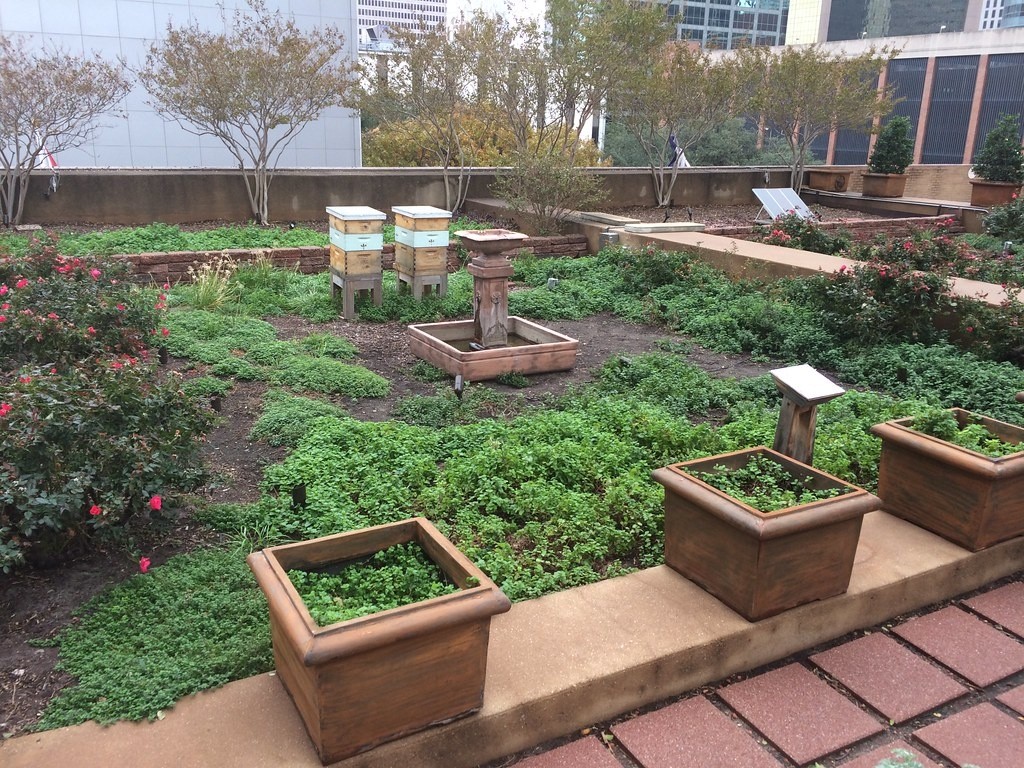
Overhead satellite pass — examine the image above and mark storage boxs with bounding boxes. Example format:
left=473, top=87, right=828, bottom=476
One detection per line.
left=394, top=213, right=448, bottom=277
left=328, top=214, right=385, bottom=279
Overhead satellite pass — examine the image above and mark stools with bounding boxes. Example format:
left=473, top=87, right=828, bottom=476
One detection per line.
left=329, top=267, right=382, bottom=320
left=393, top=263, right=448, bottom=302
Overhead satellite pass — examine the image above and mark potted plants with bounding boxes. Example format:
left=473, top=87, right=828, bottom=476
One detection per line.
left=649, top=446, right=887, bottom=623
left=246, top=516, right=510, bottom=766
left=968, top=113, right=1024, bottom=207
left=870, top=407, right=1024, bottom=552
left=862, top=116, right=914, bottom=197
